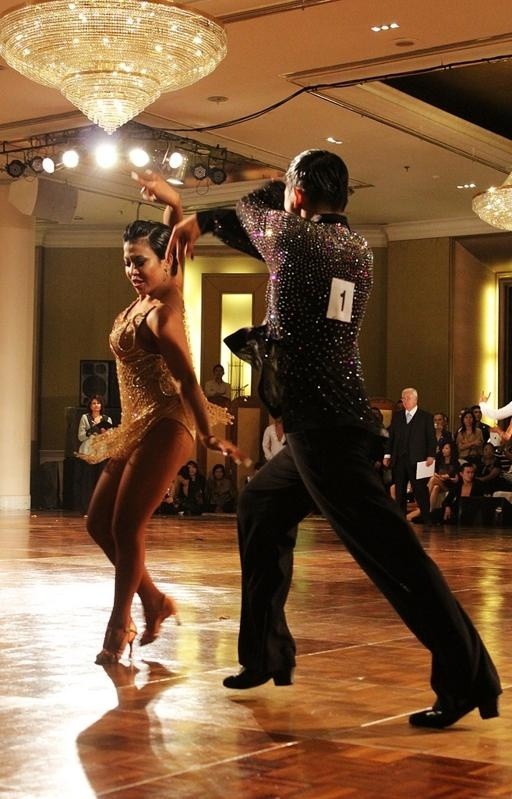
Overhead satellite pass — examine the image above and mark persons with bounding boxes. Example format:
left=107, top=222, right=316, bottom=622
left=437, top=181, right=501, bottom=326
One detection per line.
left=262, top=418, right=287, bottom=462
left=165, top=148, right=502, bottom=730
left=368, top=388, right=512, bottom=530
left=78, top=394, right=114, bottom=443
left=201, top=365, right=228, bottom=408
left=85, top=158, right=229, bottom=664
left=153, top=460, right=233, bottom=520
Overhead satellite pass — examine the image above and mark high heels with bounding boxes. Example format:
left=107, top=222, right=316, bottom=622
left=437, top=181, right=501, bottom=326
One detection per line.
left=95, top=594, right=182, bottom=664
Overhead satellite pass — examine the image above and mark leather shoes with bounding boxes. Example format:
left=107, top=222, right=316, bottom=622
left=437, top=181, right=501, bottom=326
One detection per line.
left=223, top=664, right=295, bottom=689
left=410, top=694, right=499, bottom=728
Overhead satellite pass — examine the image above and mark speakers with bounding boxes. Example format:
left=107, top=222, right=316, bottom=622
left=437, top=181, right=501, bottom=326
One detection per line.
left=79, top=359, right=120, bottom=408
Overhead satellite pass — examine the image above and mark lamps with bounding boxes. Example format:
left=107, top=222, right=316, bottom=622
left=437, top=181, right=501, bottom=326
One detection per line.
left=0, top=121, right=226, bottom=195
left=471, top=171, right=512, bottom=231
left=0, top=0, right=227, bottom=135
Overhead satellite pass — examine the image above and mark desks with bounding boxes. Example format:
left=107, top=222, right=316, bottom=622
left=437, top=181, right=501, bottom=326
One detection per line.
left=40, top=450, right=65, bottom=508
left=458, top=496, right=512, bottom=529
